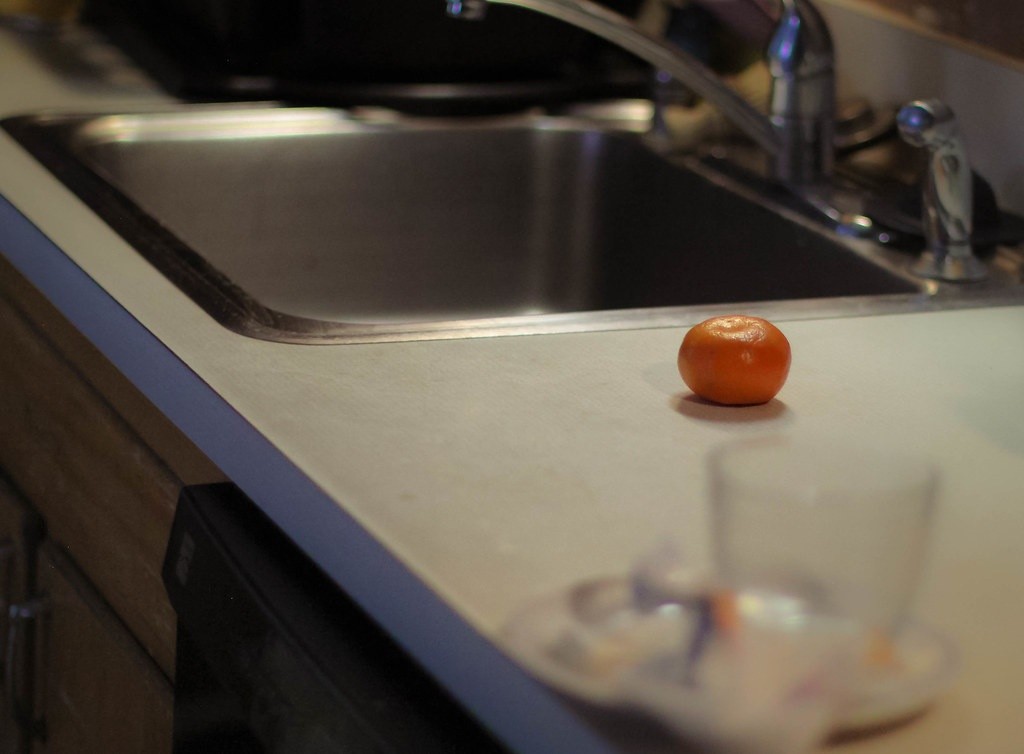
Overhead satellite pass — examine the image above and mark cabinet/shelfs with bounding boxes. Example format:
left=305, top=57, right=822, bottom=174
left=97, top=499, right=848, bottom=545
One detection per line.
left=0, top=254, right=515, bottom=754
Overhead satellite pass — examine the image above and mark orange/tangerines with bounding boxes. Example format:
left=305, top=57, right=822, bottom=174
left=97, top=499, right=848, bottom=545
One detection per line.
left=677, top=314, right=792, bottom=406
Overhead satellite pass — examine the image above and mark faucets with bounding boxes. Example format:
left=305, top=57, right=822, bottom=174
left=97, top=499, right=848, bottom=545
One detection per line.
left=439, top=0, right=849, bottom=205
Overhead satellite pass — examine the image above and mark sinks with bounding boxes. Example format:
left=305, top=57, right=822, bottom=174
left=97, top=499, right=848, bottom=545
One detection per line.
left=0, top=107, right=946, bottom=346
